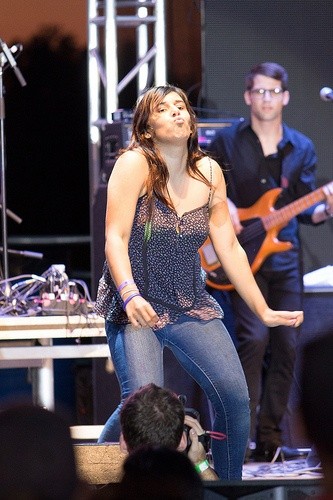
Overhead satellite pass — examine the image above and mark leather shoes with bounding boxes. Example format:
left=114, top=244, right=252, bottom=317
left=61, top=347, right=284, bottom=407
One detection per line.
left=251, top=445, right=303, bottom=461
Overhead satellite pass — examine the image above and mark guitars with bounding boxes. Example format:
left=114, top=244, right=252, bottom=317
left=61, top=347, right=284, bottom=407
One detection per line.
left=197, top=180, right=333, bottom=292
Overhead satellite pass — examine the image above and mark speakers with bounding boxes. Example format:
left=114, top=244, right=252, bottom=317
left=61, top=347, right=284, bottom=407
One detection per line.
left=288, top=285, right=333, bottom=453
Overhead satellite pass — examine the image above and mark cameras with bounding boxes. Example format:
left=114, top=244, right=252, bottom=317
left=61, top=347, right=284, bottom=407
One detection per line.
left=178, top=395, right=201, bottom=457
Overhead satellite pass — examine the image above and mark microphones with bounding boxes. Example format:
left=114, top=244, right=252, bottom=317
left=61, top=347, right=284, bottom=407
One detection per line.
left=320, top=87, right=333, bottom=102
left=0, top=43, right=21, bottom=67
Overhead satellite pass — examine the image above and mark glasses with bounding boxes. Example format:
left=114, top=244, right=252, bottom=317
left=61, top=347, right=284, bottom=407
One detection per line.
left=249, top=87, right=284, bottom=94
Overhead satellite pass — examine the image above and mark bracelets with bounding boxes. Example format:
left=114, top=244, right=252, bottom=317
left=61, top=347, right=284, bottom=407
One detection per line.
left=122, top=292, right=143, bottom=312
left=120, top=288, right=140, bottom=299
left=324, top=204, right=333, bottom=218
left=117, top=280, right=137, bottom=293
left=192, top=460, right=210, bottom=474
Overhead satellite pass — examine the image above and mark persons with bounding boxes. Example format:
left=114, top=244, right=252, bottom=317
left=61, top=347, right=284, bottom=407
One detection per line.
left=0, top=328, right=333, bottom=500
left=207, top=63, right=333, bottom=464
left=91, top=85, right=304, bottom=480
